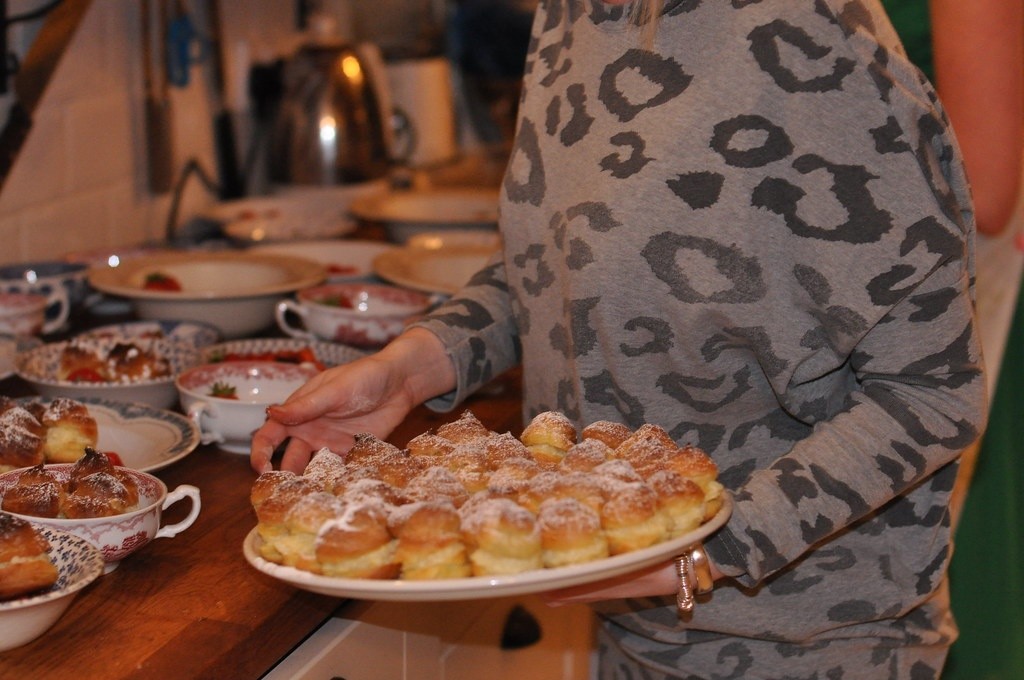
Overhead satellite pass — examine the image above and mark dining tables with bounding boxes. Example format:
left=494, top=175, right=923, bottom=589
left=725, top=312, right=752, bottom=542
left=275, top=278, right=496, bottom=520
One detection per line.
left=0, top=294, right=598, bottom=680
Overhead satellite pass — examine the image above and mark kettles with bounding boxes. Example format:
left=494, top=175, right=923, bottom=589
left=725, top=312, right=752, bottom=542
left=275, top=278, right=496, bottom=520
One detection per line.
left=277, top=37, right=390, bottom=188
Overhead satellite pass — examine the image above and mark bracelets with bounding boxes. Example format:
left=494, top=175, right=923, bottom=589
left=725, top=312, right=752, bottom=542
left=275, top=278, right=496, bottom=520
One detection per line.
left=673, top=556, right=695, bottom=611
left=689, top=545, right=713, bottom=595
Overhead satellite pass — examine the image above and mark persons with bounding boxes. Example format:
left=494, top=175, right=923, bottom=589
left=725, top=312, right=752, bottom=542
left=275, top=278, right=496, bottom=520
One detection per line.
left=251, top=0, right=986, bottom=679
left=879, top=0, right=1024, bottom=680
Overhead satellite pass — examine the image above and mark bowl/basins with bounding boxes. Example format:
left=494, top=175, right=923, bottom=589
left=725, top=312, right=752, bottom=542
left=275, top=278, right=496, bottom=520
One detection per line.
left=12, top=395, right=202, bottom=473
left=347, top=186, right=499, bottom=245
left=274, top=282, right=430, bottom=351
left=0, top=261, right=90, bottom=338
left=199, top=338, right=370, bottom=372
left=76, top=318, right=221, bottom=348
left=0, top=521, right=105, bottom=653
left=0, top=463, right=202, bottom=576
left=196, top=180, right=390, bottom=243
left=11, top=336, right=201, bottom=411
left=86, top=249, right=330, bottom=340
left=172, top=361, right=319, bottom=455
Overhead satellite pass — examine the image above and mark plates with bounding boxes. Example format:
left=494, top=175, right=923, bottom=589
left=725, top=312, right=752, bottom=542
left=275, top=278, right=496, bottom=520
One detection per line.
left=372, top=243, right=500, bottom=296
left=242, top=493, right=731, bottom=601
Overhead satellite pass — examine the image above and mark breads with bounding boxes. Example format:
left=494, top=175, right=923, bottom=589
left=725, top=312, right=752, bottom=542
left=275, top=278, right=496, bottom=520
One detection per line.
left=0, top=396, right=151, bottom=600
left=249, top=410, right=724, bottom=581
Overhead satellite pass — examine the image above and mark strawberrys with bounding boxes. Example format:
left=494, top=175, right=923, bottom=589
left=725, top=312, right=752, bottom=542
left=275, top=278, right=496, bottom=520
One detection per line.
left=142, top=272, right=181, bottom=291
left=207, top=382, right=239, bottom=400
left=65, top=369, right=104, bottom=383
